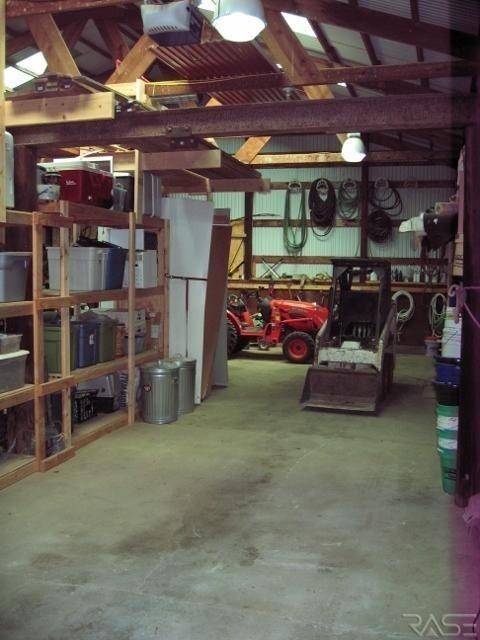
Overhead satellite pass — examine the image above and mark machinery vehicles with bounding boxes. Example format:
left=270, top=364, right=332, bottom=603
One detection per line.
left=227, top=257, right=399, bottom=412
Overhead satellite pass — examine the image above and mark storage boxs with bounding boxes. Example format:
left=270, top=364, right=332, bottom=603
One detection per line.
left=0, top=333, right=23, bottom=354
left=36, top=161, right=134, bottom=212
left=44, top=308, right=157, bottom=373
left=76, top=373, right=122, bottom=414
left=46, top=247, right=158, bottom=291
left=0, top=252, right=32, bottom=302
left=0, top=350, right=30, bottom=393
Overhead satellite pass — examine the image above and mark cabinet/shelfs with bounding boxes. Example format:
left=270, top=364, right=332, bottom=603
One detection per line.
left=0, top=210, right=169, bottom=490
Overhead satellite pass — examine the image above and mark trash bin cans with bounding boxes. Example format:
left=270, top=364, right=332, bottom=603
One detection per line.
left=139, top=361, right=179, bottom=425
left=159, top=358, right=197, bottom=414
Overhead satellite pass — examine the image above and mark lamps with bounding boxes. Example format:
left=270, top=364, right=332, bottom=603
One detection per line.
left=211, top=0, right=267, bottom=43
left=341, top=133, right=367, bottom=163
